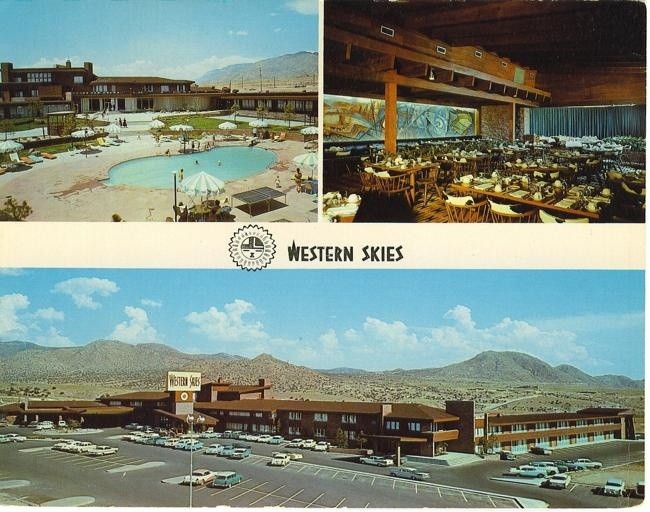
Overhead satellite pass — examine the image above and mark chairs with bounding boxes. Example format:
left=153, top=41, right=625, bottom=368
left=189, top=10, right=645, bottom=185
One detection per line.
left=275, top=132, right=286, bottom=142
left=97, top=136, right=120, bottom=147
left=334, top=145, right=646, bottom=223
left=172, top=205, right=231, bottom=222
left=9, top=152, right=57, bottom=166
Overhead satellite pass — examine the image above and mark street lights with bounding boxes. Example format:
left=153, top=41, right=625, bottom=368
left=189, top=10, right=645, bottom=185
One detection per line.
left=171, top=170, right=178, bottom=222
left=84, top=128, right=88, bottom=157
left=186, top=414, right=205, bottom=507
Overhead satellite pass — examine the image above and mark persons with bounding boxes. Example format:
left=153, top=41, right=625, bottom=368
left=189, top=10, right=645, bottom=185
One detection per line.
left=275, top=176, right=282, bottom=188
left=294, top=168, right=302, bottom=193
left=179, top=201, right=188, bottom=213
left=253, top=128, right=258, bottom=136
left=178, top=169, right=184, bottom=184
left=209, top=200, right=221, bottom=221
left=164, top=149, right=170, bottom=157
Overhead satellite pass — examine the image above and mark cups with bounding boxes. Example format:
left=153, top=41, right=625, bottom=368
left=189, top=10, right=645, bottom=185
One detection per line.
left=461, top=171, right=612, bottom=210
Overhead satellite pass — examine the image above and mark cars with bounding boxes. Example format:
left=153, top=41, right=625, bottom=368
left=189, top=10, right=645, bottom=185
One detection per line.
left=495, top=450, right=516, bottom=460
left=200, top=431, right=331, bottom=466
left=604, top=479, right=625, bottom=496
left=184, top=468, right=241, bottom=488
left=29, top=421, right=66, bottom=430
left=54, top=440, right=118, bottom=456
left=0, top=433, right=26, bottom=443
left=123, top=423, right=204, bottom=451
left=531, top=446, right=552, bottom=455
left=508, top=458, right=602, bottom=488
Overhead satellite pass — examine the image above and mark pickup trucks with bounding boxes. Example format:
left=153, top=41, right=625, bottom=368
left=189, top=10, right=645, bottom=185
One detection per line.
left=359, top=456, right=394, bottom=467
left=390, top=468, right=430, bottom=480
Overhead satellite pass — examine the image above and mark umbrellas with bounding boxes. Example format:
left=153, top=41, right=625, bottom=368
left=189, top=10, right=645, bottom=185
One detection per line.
left=218, top=121, right=238, bottom=137
left=302, top=127, right=318, bottom=142
left=177, top=170, right=226, bottom=222
left=168, top=124, right=194, bottom=133
left=293, top=152, right=318, bottom=180
left=0, top=140, right=25, bottom=154
left=70, top=129, right=96, bottom=153
left=149, top=119, right=166, bottom=130
left=104, top=123, right=121, bottom=142
left=249, top=118, right=271, bottom=130
